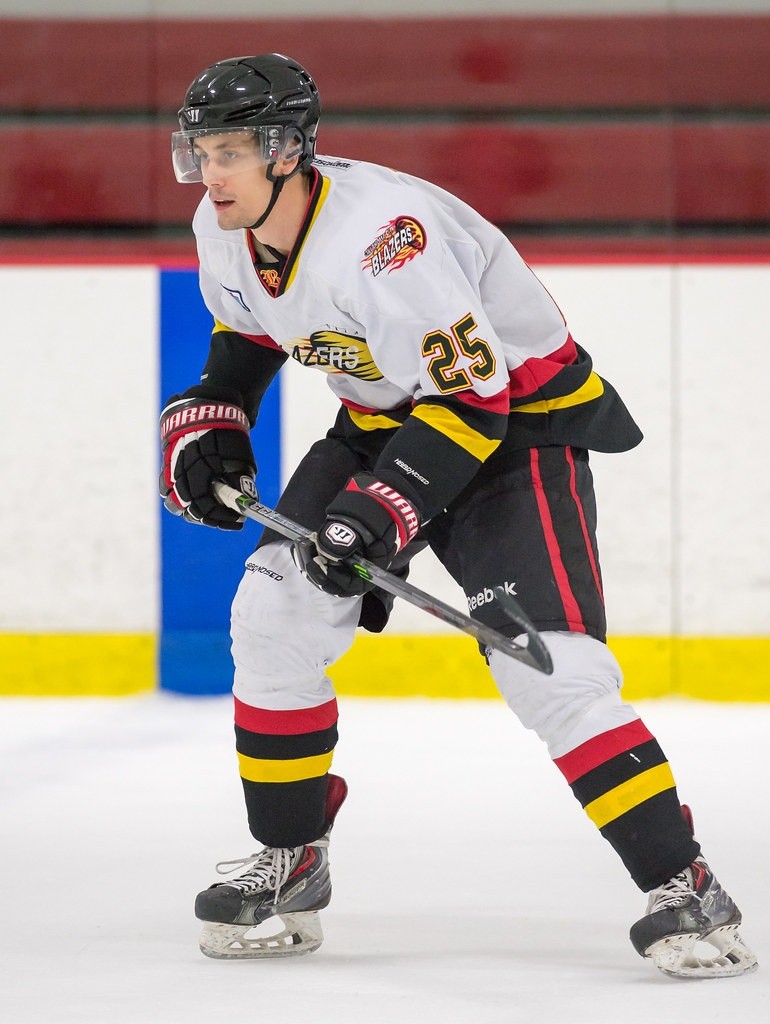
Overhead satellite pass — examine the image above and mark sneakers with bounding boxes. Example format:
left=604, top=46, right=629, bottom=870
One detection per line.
left=627, top=856, right=760, bottom=979
left=195, top=846, right=333, bottom=960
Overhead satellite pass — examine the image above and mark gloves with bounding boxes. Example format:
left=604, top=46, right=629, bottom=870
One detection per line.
left=289, top=470, right=423, bottom=599
left=159, top=385, right=260, bottom=532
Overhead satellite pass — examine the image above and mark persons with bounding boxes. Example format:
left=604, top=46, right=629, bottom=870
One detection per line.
left=157, top=54, right=759, bottom=981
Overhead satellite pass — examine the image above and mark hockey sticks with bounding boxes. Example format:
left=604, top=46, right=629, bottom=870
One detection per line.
left=213, top=482, right=557, bottom=680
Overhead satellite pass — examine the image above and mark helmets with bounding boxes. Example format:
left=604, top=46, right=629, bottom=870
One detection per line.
left=178, top=53, right=323, bottom=184
